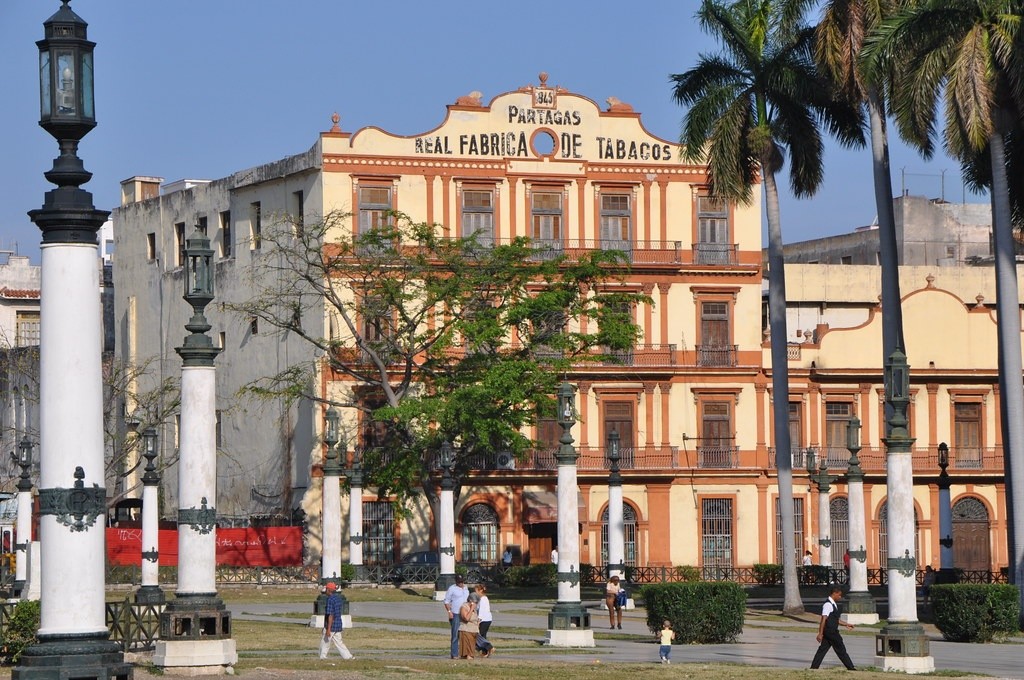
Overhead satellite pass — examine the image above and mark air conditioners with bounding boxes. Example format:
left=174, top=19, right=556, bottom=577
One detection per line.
left=496, top=451, right=515, bottom=469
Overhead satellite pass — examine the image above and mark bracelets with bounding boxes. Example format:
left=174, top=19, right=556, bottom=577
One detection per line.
left=448, top=610, right=451, bottom=613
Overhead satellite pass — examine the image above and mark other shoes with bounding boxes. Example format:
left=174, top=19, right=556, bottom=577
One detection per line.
left=451, top=655, right=459, bottom=660
left=617, top=623, right=623, bottom=630
left=610, top=625, right=614, bottom=629
left=661, top=657, right=667, bottom=664
left=487, top=647, right=496, bottom=657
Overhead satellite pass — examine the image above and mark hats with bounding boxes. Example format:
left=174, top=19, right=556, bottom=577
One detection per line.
left=455, top=574, right=466, bottom=583
left=327, top=583, right=336, bottom=591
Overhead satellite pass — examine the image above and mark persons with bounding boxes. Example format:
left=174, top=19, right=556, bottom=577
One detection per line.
left=809, top=586, right=857, bottom=671
left=503, top=545, right=513, bottom=566
left=921, top=565, right=936, bottom=608
left=657, top=620, right=676, bottom=664
left=550, top=545, right=559, bottom=565
left=802, top=551, right=813, bottom=565
left=319, top=582, right=356, bottom=660
left=843, top=549, right=850, bottom=585
left=443, top=574, right=496, bottom=659
left=606, top=575, right=623, bottom=629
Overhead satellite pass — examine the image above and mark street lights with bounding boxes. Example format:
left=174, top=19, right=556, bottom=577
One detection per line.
left=309, top=402, right=352, bottom=629
left=541, top=371, right=596, bottom=647
left=11, top=0, right=134, bottom=680
left=873, top=334, right=935, bottom=676
left=839, top=414, right=880, bottom=624
left=600, top=427, right=634, bottom=610
left=936, top=442, right=957, bottom=585
left=135, top=419, right=166, bottom=606
left=433, top=437, right=456, bottom=601
left=152, top=224, right=240, bottom=667
left=7, top=436, right=34, bottom=598
